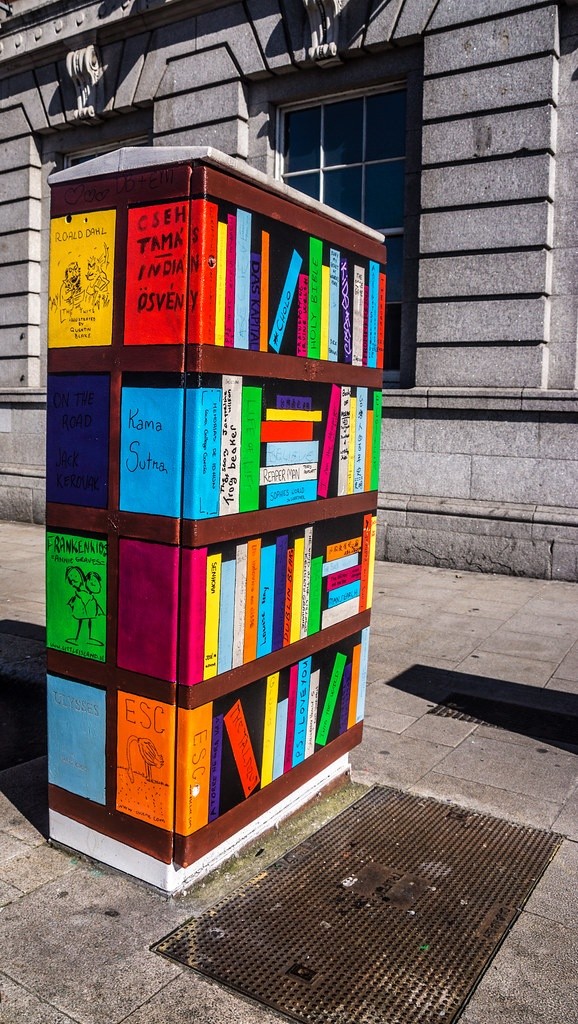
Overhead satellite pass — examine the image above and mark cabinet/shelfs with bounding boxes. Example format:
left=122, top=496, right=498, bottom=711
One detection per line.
left=47, top=145, right=386, bottom=896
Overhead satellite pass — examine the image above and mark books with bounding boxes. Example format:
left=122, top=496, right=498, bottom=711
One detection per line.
left=46, top=514, right=378, bottom=685
left=48, top=627, right=370, bottom=836
left=47, top=374, right=383, bottom=520
left=49, top=196, right=387, bottom=368
